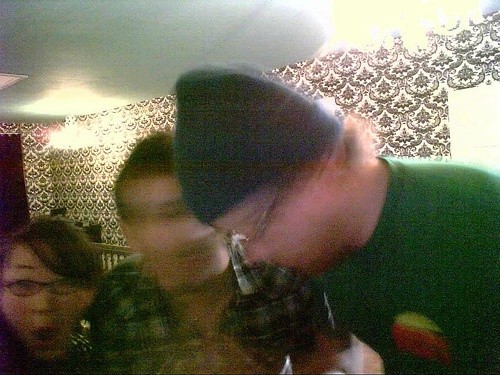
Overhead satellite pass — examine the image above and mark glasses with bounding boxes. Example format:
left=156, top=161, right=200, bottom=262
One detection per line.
left=3, top=276, right=88, bottom=297
left=222, top=186, right=287, bottom=256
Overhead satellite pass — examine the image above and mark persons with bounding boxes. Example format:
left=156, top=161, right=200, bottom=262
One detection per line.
left=88, top=129, right=366, bottom=375
left=174, top=66, right=499, bottom=374
left=1, top=218, right=102, bottom=375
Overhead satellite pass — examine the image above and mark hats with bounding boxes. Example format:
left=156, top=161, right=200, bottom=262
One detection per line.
left=175, top=66, right=341, bottom=224
